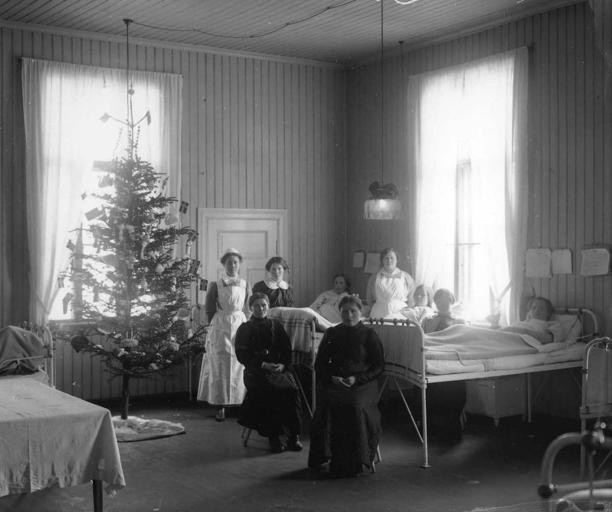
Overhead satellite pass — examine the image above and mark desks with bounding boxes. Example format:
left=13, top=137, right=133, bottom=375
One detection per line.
left=0, top=376, right=126, bottom=512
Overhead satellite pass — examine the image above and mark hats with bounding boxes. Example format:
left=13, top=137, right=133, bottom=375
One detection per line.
left=220, top=248, right=243, bottom=264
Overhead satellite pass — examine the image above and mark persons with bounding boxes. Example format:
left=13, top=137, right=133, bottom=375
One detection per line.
left=251, top=256, right=294, bottom=308
left=308, top=273, right=353, bottom=322
left=306, top=294, right=385, bottom=478
left=194, top=247, right=253, bottom=421
left=401, top=281, right=434, bottom=322
left=364, top=247, right=414, bottom=318
left=496, top=295, right=564, bottom=344
left=414, top=289, right=467, bottom=446
left=234, top=292, right=305, bottom=454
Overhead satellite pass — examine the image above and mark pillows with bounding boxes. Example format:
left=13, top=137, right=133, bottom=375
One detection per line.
left=547, top=313, right=581, bottom=347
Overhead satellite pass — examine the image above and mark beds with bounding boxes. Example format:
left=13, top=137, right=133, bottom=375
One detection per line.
left=268, top=307, right=344, bottom=421
left=0, top=321, right=54, bottom=389
left=357, top=306, right=598, bottom=470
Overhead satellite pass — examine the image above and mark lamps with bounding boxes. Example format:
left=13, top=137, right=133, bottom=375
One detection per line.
left=365, top=0, right=403, bottom=221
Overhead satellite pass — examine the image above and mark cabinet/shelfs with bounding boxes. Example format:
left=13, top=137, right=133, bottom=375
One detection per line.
left=465, top=374, right=529, bottom=429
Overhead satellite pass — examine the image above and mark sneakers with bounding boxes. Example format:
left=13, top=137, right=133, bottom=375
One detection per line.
left=269, top=437, right=286, bottom=452
left=287, top=438, right=302, bottom=451
left=216, top=410, right=226, bottom=421
